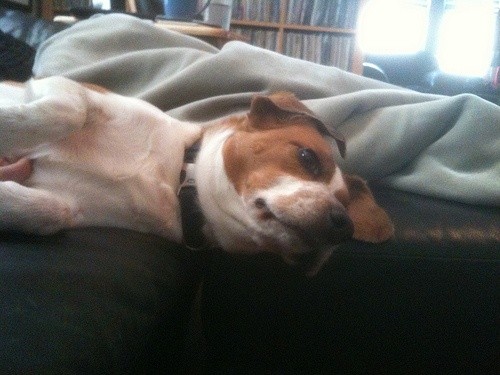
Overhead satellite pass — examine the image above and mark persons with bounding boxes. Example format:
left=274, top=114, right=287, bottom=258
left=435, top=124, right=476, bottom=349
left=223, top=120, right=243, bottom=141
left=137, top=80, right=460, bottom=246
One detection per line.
left=0, top=0, right=77, bottom=184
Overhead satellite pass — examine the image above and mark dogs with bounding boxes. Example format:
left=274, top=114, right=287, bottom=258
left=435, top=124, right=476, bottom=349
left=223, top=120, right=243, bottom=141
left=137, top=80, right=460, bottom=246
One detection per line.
left=0, top=75, right=395, bottom=256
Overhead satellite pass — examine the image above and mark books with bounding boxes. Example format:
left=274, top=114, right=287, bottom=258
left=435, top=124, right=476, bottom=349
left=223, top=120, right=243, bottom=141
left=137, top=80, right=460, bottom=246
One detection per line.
left=230, top=0, right=360, bottom=71
